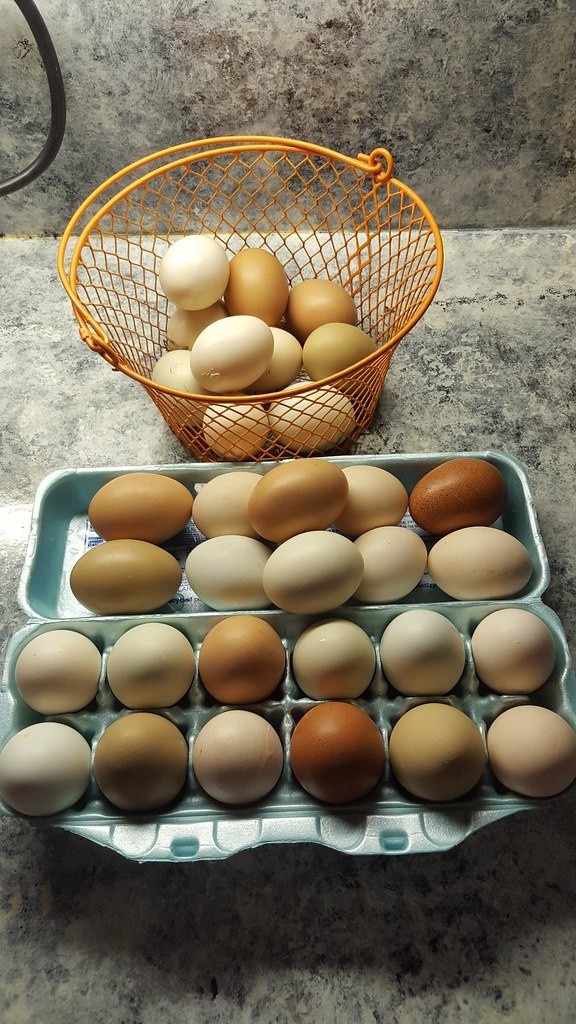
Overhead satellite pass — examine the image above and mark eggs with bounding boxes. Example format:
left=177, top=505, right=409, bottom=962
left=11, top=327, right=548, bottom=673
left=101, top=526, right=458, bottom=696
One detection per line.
left=0, top=722, right=92, bottom=816
left=486, top=704, right=576, bottom=798
left=199, top=615, right=286, bottom=706
left=107, top=622, right=197, bottom=709
left=388, top=702, right=486, bottom=803
left=15, top=629, right=102, bottom=716
left=150, top=236, right=379, bottom=461
left=289, top=701, right=386, bottom=804
left=193, top=710, right=283, bottom=806
left=94, top=713, right=188, bottom=812
left=379, top=610, right=466, bottom=696
left=292, top=618, right=376, bottom=701
left=471, top=608, right=557, bottom=696
left=70, top=456, right=534, bottom=615
